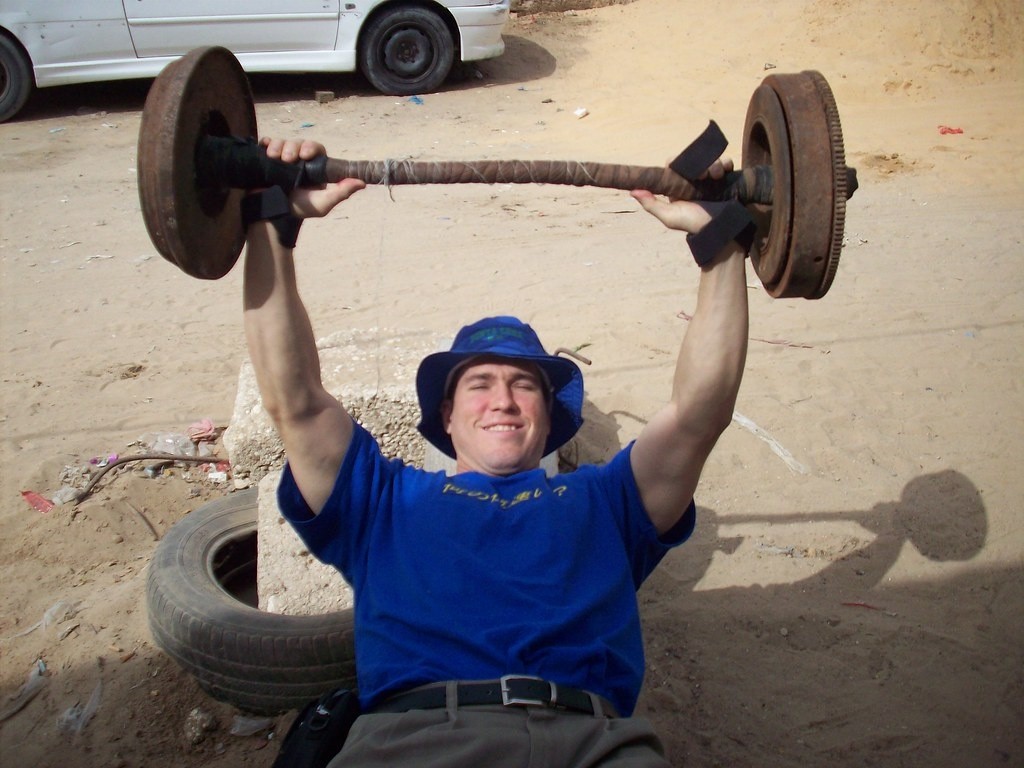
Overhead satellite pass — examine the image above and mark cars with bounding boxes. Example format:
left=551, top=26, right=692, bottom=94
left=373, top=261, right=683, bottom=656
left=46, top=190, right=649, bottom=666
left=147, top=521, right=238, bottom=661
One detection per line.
left=0, top=0, right=512, bottom=131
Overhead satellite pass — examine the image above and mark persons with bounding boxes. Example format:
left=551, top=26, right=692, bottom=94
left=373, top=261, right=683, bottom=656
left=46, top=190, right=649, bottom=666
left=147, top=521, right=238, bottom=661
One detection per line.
left=242, top=138, right=750, bottom=768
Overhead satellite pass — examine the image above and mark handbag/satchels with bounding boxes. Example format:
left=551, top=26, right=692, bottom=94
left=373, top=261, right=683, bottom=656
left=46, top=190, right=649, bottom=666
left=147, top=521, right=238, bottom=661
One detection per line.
left=271, top=687, right=363, bottom=768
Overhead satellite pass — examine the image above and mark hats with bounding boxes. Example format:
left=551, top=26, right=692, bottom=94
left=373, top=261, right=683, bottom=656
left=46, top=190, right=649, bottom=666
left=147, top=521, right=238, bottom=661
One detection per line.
left=415, top=316, right=585, bottom=460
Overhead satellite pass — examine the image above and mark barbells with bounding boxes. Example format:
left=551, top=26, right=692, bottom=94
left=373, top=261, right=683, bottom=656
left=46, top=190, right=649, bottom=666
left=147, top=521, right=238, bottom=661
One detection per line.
left=122, top=38, right=874, bottom=309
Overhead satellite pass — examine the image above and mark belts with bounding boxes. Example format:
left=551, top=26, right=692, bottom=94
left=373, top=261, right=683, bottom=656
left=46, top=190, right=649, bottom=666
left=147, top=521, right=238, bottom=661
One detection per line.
left=362, top=674, right=621, bottom=719
